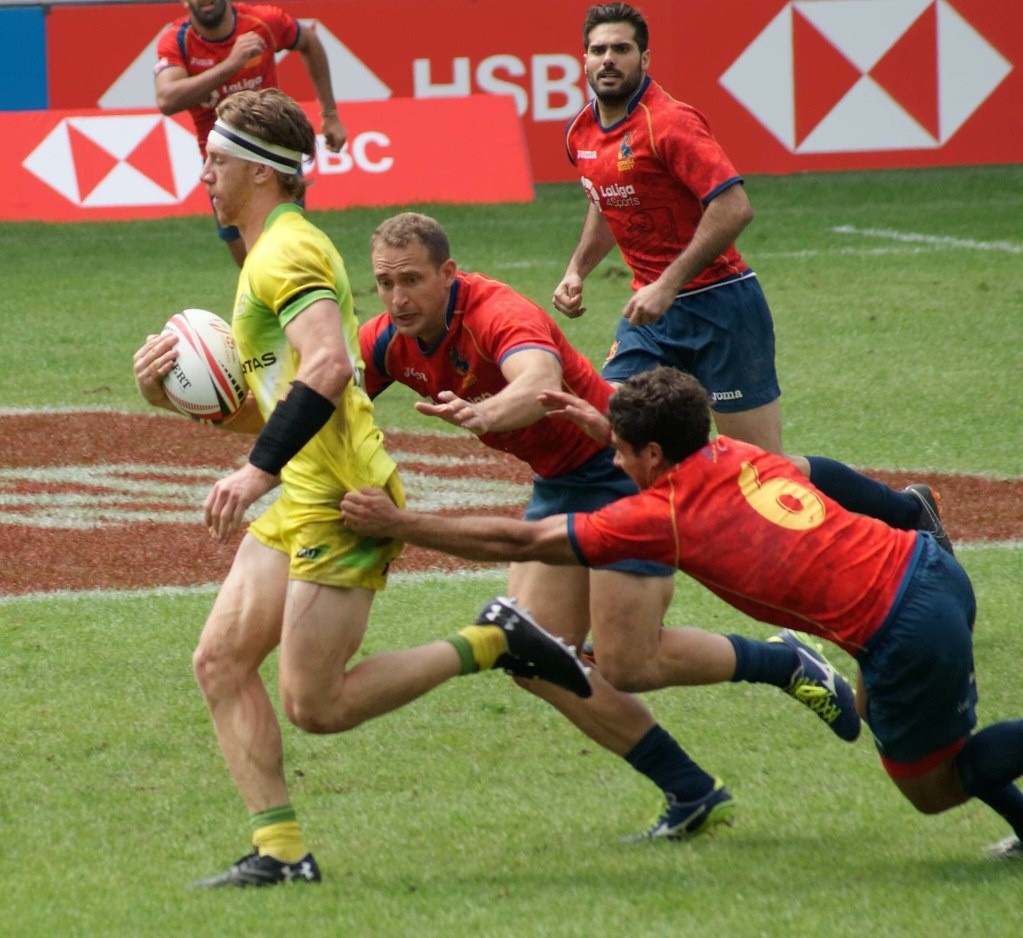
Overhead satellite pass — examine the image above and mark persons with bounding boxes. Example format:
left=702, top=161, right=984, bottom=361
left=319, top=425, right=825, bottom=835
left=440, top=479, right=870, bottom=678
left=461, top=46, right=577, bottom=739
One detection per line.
left=154, top=0, right=348, bottom=269
left=552, top=0, right=955, bottom=557
left=341, top=366, right=1023, bottom=858
left=358, top=213, right=862, bottom=844
left=134, top=87, right=596, bottom=889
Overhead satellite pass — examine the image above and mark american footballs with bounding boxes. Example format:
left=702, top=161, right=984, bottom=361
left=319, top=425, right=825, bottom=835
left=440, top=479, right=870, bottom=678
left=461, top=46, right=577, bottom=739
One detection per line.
left=157, top=308, right=251, bottom=426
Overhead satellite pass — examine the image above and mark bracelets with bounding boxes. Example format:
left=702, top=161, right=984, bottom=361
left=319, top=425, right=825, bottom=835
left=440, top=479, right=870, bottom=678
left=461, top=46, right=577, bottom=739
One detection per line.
left=321, top=109, right=337, bottom=115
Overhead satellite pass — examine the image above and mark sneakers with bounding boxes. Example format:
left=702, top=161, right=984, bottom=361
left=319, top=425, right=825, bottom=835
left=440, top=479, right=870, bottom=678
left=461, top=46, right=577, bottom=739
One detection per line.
left=986, top=833, right=1023, bottom=863
left=472, top=595, right=593, bottom=699
left=766, top=628, right=862, bottom=745
left=628, top=775, right=737, bottom=846
left=900, top=483, right=955, bottom=558
left=192, top=850, right=322, bottom=890
